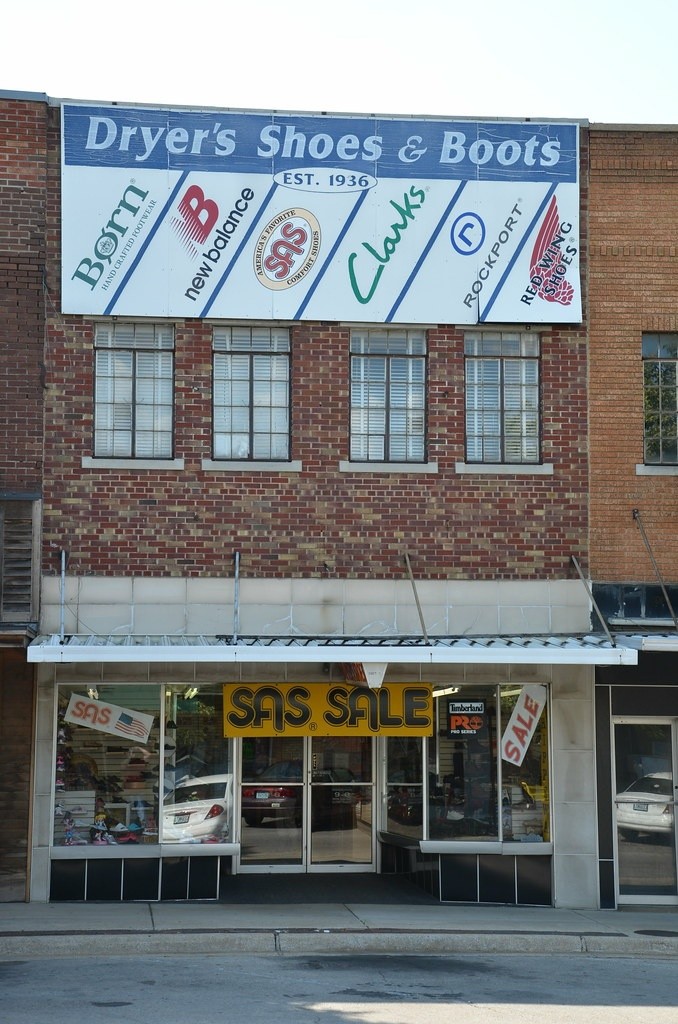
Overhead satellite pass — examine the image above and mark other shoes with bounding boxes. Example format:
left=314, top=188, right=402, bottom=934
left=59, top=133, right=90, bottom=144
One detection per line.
left=128, top=758, right=149, bottom=764
left=107, top=746, right=129, bottom=752
left=112, top=795, right=128, bottom=803
left=55, top=800, right=159, bottom=845
left=152, top=763, right=177, bottom=771
left=153, top=786, right=173, bottom=793
left=95, top=798, right=105, bottom=812
left=126, top=776, right=145, bottom=782
left=96, top=775, right=124, bottom=792
left=57, top=765, right=66, bottom=771
left=167, top=720, right=177, bottom=728
left=139, top=771, right=159, bottom=778
left=55, top=779, right=64, bottom=786
left=57, top=755, right=65, bottom=764
left=83, top=740, right=103, bottom=747
left=154, top=743, right=175, bottom=750
left=59, top=708, right=67, bottom=717
left=432, top=729, right=536, bottom=821
left=134, top=801, right=153, bottom=807
left=57, top=739, right=66, bottom=745
left=57, top=728, right=65, bottom=737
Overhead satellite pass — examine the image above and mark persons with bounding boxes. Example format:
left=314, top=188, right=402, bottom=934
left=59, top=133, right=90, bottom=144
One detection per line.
left=121, top=746, right=151, bottom=826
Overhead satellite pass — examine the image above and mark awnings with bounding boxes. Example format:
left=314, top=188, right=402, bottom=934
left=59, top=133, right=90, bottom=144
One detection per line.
left=27, top=514, right=678, bottom=665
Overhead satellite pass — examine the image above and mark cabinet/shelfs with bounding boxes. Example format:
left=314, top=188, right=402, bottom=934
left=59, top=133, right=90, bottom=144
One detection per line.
left=53, top=683, right=177, bottom=846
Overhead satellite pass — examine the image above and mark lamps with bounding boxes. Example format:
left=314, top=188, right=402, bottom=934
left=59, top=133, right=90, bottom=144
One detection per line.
left=184, top=685, right=200, bottom=699
left=432, top=685, right=462, bottom=697
left=87, top=686, right=98, bottom=700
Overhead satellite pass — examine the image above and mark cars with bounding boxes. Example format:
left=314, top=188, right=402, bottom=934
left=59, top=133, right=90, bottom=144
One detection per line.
left=616, top=772, right=675, bottom=841
left=242, top=761, right=367, bottom=828
left=163, top=773, right=233, bottom=844
left=388, top=779, right=544, bottom=839
